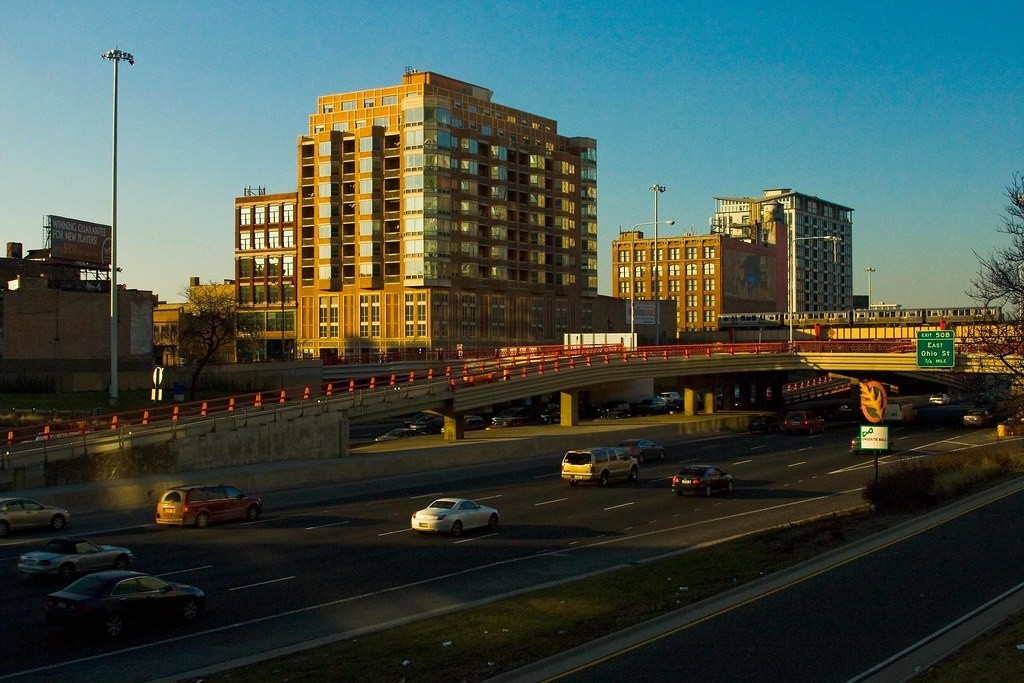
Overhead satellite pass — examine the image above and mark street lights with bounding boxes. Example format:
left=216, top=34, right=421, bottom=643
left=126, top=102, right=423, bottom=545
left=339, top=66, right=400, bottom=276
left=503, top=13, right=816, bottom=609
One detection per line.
left=100, top=43, right=132, bottom=416
left=866, top=267, right=876, bottom=313
left=651, top=184, right=665, bottom=349
left=628, top=220, right=675, bottom=348
left=788, top=235, right=839, bottom=348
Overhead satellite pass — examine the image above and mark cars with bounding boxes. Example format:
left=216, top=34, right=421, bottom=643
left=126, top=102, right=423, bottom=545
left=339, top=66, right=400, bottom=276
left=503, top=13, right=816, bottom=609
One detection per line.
left=560, top=446, right=638, bottom=486
left=41, top=569, right=207, bottom=638
left=374, top=390, right=719, bottom=441
left=0, top=496, right=69, bottom=533
left=961, top=409, right=992, bottom=427
left=17, top=536, right=135, bottom=580
left=851, top=435, right=893, bottom=455
left=153, top=482, right=263, bottom=527
left=882, top=403, right=919, bottom=424
left=781, top=411, right=825, bottom=433
left=615, top=439, right=664, bottom=465
left=412, top=498, right=498, bottom=536
left=670, top=465, right=733, bottom=496
left=929, top=392, right=950, bottom=405
